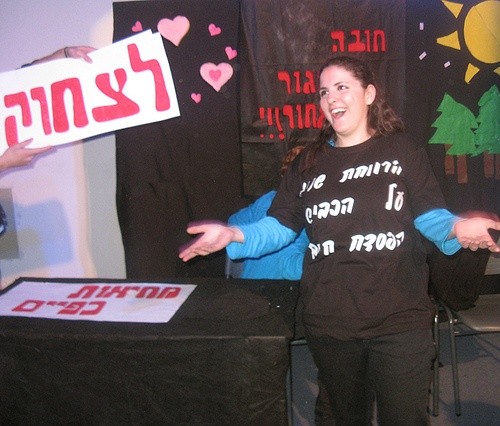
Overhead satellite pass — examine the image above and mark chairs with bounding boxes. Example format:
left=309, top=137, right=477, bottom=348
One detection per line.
left=427, top=229, right=500, bottom=416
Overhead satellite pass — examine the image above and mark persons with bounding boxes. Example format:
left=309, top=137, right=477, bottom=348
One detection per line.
left=224, top=146, right=316, bottom=280
left=179, top=57, right=499, bottom=426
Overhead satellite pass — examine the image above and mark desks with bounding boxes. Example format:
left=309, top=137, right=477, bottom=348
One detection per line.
left=0, top=277, right=300, bottom=426
